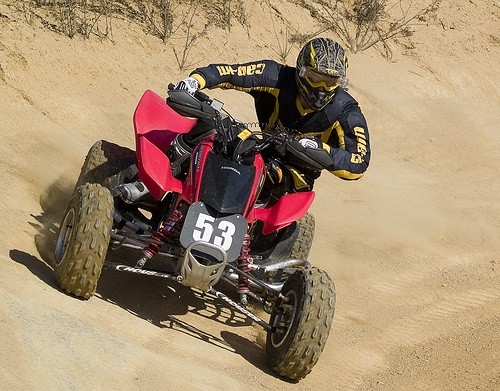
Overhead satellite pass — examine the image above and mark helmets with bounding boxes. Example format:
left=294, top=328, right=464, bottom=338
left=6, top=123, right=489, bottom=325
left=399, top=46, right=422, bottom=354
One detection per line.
left=294, top=37, right=349, bottom=112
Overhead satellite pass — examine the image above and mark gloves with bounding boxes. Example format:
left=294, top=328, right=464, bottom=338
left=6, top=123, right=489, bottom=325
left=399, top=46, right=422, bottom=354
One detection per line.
left=298, top=138, right=319, bottom=150
left=173, top=76, right=199, bottom=96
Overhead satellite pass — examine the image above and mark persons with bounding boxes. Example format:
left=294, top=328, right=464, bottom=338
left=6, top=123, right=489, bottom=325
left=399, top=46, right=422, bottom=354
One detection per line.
left=116, top=38, right=371, bottom=209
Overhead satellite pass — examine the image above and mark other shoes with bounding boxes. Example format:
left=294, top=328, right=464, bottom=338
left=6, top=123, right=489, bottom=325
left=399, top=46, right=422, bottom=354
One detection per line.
left=114, top=179, right=158, bottom=207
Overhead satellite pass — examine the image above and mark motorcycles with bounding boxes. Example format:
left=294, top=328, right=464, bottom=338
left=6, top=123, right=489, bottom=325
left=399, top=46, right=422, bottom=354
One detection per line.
left=55, top=82, right=336, bottom=384
